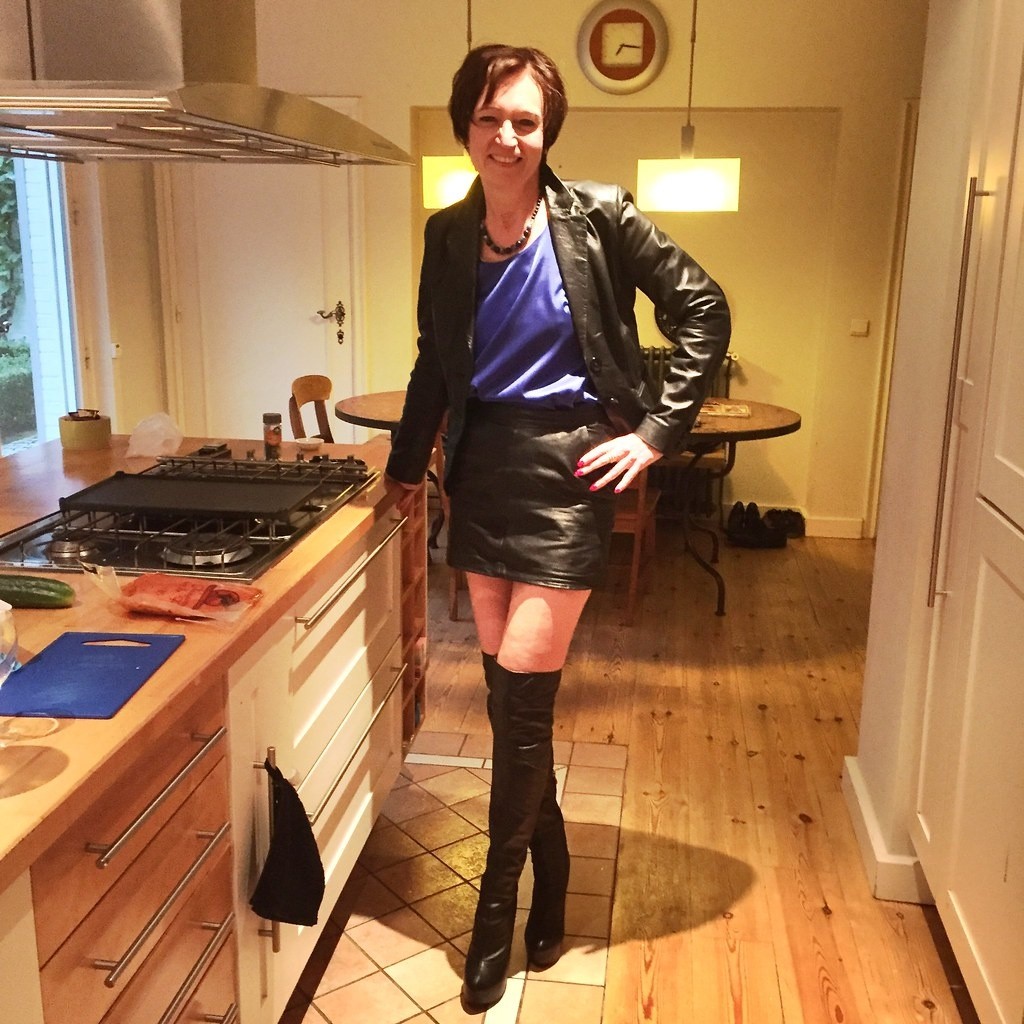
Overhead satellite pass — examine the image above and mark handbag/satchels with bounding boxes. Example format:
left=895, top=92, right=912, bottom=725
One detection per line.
left=250, top=760, right=325, bottom=927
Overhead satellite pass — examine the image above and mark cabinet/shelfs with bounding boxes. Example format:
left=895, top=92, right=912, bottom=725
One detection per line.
left=0, top=472, right=429, bottom=1024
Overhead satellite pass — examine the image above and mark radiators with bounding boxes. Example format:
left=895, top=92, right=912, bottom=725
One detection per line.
left=639, top=346, right=738, bottom=517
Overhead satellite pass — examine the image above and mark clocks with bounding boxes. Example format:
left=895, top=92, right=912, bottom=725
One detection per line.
left=577, top=0, right=666, bottom=92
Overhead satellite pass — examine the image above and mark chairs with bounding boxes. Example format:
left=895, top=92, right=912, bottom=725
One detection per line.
left=288, top=374, right=442, bottom=566
left=589, top=447, right=663, bottom=623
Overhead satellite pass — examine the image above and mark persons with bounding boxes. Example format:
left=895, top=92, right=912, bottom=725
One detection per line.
left=382, top=42, right=732, bottom=1007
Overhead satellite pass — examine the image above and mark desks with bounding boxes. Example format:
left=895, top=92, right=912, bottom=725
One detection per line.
left=335, top=390, right=799, bottom=615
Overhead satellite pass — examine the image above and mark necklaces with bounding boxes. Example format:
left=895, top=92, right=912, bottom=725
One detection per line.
left=481, top=184, right=542, bottom=255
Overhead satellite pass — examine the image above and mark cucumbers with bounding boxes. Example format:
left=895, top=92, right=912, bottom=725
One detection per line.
left=0, top=574, right=76, bottom=608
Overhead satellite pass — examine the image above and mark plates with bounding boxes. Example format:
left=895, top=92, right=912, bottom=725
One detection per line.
left=296, top=438, right=325, bottom=450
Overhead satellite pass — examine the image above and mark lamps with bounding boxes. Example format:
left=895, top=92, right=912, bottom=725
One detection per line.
left=423, top=0, right=487, bottom=208
left=636, top=0, right=742, bottom=212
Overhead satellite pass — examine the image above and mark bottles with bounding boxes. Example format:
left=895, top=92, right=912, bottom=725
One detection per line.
left=263, top=413, right=283, bottom=460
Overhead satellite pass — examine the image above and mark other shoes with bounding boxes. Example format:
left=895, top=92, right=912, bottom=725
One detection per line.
left=784, top=510, right=804, bottom=531
left=768, top=509, right=781, bottom=524
left=726, top=518, right=788, bottom=548
left=744, top=502, right=759, bottom=523
left=730, top=501, right=744, bottom=524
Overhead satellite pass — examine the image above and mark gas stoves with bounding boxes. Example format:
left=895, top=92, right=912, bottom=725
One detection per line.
left=0, top=441, right=383, bottom=584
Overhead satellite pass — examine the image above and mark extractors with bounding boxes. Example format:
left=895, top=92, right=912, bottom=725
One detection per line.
left=0, top=0, right=420, bottom=168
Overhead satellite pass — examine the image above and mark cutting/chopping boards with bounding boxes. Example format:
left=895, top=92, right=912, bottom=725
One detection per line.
left=0, top=632, right=185, bottom=719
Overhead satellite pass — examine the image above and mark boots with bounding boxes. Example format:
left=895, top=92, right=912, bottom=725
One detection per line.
left=464, top=654, right=562, bottom=1012
left=482, top=650, right=570, bottom=970
